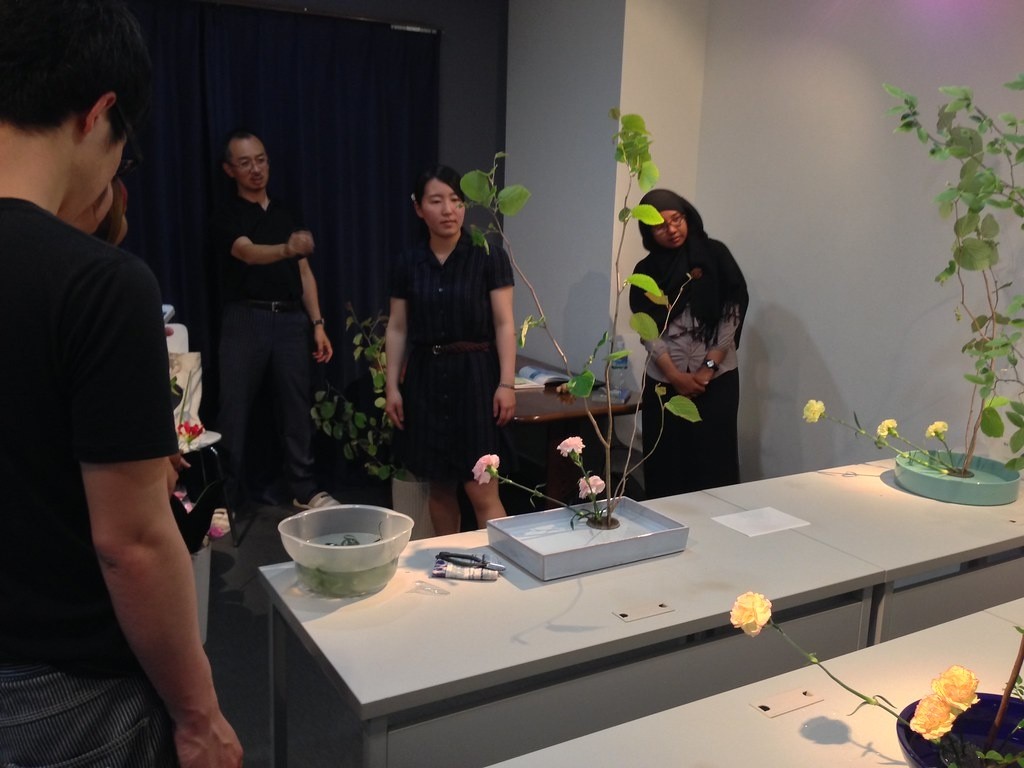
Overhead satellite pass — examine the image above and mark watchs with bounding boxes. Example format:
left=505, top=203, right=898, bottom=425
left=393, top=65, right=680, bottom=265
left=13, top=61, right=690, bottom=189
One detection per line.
left=310, top=318, right=324, bottom=325
left=703, top=359, right=719, bottom=371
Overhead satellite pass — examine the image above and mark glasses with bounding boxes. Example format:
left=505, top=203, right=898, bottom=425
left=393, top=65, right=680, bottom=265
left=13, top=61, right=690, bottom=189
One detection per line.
left=231, top=159, right=268, bottom=168
left=654, top=215, right=686, bottom=233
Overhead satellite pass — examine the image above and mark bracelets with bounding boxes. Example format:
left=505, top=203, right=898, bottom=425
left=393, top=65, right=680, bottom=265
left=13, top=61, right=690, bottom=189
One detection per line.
left=498, top=383, right=515, bottom=389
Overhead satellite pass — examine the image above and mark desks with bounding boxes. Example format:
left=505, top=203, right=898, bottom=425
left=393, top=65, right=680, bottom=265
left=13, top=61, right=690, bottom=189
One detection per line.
left=485, top=598, right=1024, bottom=768
left=496, top=346, right=643, bottom=506
left=254, top=495, right=883, bottom=768
left=704, top=446, right=1024, bottom=646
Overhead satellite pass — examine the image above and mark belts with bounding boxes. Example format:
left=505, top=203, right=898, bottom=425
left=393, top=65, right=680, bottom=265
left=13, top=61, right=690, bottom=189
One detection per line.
left=246, top=301, right=298, bottom=313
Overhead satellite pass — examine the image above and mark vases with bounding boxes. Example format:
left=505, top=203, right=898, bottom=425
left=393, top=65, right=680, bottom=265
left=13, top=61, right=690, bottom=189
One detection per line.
left=890, top=449, right=1020, bottom=505
left=897, top=692, right=1024, bottom=768
left=489, top=496, right=692, bottom=583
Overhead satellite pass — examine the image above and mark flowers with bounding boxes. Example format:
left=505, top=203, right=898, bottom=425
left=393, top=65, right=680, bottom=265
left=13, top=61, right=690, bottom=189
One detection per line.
left=729, top=590, right=1024, bottom=768
left=421, top=110, right=692, bottom=529
left=802, top=73, right=1024, bottom=476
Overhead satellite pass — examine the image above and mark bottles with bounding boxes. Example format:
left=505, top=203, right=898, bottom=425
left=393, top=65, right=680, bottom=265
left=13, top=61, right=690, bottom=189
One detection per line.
left=611, top=341, right=628, bottom=391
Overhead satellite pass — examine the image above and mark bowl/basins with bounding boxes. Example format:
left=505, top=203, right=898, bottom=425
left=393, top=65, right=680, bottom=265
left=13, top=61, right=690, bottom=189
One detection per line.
left=278, top=504, right=415, bottom=598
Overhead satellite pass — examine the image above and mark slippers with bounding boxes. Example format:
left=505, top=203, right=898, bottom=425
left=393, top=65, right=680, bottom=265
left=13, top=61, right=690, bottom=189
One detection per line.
left=210, top=509, right=235, bottom=537
left=293, top=491, right=341, bottom=510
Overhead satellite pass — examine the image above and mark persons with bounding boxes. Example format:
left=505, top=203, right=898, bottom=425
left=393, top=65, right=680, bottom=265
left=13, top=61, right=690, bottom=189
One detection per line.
left=384, top=160, right=518, bottom=536
left=629, top=188, right=750, bottom=501
left=208, top=129, right=334, bottom=539
left=0, top=0, right=245, bottom=768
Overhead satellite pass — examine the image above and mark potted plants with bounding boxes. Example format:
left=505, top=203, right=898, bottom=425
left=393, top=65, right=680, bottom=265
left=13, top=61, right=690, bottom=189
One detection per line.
left=314, top=300, right=465, bottom=538
